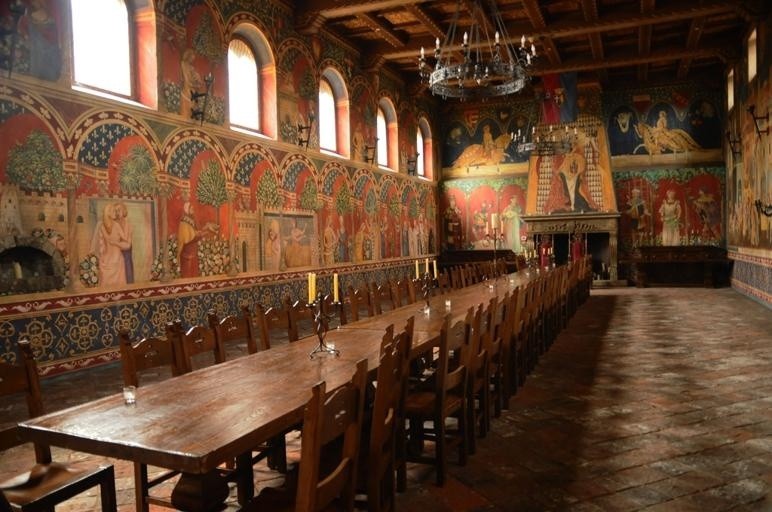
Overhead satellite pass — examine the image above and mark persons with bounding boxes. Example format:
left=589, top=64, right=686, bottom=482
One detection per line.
left=442, top=195, right=523, bottom=255
left=626, top=110, right=721, bottom=246
left=27, top=2, right=62, bottom=82
left=555, top=143, right=591, bottom=212
left=98, top=203, right=129, bottom=286
left=263, top=214, right=428, bottom=271
left=179, top=48, right=202, bottom=119
left=176, top=201, right=220, bottom=278
left=108, top=203, right=134, bottom=284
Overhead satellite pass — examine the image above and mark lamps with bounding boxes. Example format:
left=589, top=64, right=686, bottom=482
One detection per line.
left=510, top=94, right=579, bottom=160
left=417, top=0, right=541, bottom=105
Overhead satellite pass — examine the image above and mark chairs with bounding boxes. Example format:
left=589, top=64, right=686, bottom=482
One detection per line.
left=0, top=248, right=595, bottom=512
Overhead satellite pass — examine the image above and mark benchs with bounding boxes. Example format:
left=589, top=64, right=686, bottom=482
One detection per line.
left=616, top=245, right=734, bottom=289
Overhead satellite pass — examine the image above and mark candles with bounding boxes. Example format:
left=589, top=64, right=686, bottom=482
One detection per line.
left=491, top=214, right=499, bottom=228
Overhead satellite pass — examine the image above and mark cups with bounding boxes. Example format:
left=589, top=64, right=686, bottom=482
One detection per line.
left=445, top=300, right=450, bottom=306
left=123, top=385, right=136, bottom=404
left=423, top=306, right=430, bottom=315
left=489, top=262, right=556, bottom=288
